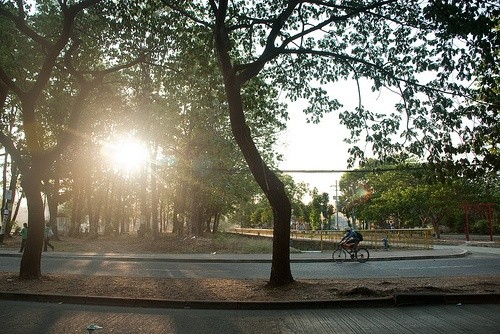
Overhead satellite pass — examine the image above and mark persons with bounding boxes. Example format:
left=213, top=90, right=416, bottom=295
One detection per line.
left=18, top=223, right=28, bottom=253
left=337, top=226, right=359, bottom=252
left=291, top=223, right=318, bottom=233
left=43, top=223, right=54, bottom=251
left=0, top=224, right=5, bottom=245
left=381, top=232, right=389, bottom=249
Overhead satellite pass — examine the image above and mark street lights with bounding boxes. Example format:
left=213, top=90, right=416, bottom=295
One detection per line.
left=329, top=179, right=338, bottom=230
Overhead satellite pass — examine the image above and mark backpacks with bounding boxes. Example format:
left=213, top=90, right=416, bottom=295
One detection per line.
left=350, top=230, right=363, bottom=241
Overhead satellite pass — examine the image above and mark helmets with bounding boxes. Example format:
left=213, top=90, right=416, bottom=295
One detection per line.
left=346, top=227, right=351, bottom=230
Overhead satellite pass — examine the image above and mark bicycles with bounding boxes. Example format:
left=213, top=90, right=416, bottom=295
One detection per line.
left=332, top=243, right=370, bottom=264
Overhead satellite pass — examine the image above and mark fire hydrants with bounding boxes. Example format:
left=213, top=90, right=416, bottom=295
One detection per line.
left=381, top=236, right=389, bottom=251
